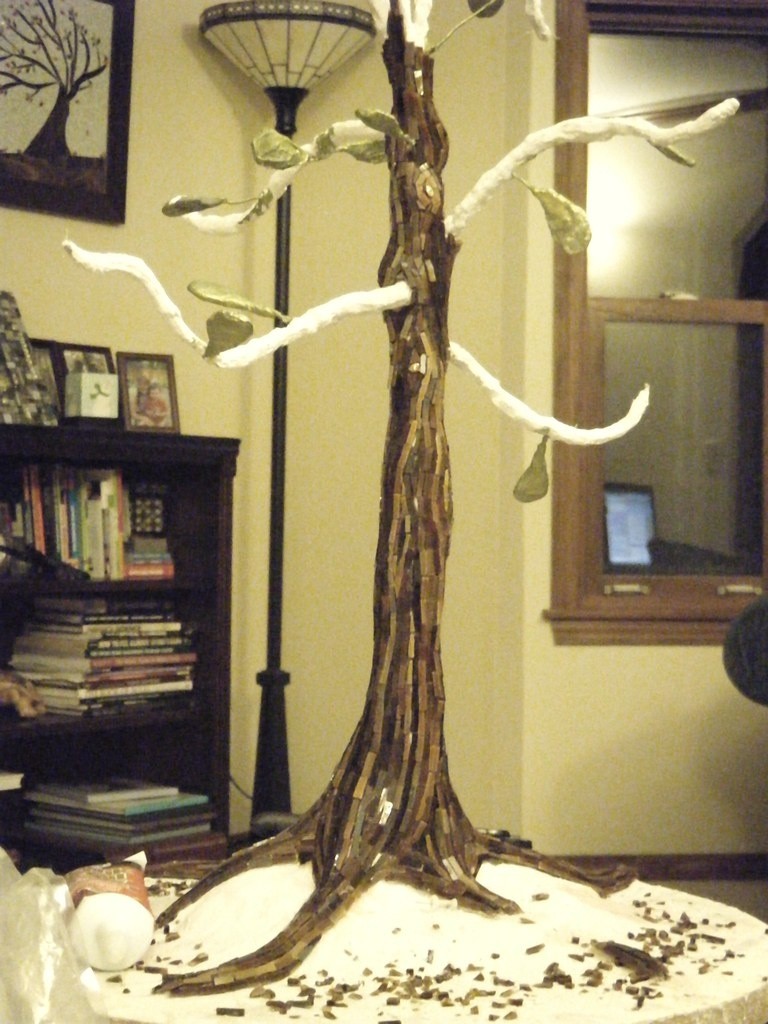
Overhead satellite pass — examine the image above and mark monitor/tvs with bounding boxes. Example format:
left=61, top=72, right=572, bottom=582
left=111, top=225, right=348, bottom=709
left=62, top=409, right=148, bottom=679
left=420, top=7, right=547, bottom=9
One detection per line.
left=602, top=484, right=659, bottom=573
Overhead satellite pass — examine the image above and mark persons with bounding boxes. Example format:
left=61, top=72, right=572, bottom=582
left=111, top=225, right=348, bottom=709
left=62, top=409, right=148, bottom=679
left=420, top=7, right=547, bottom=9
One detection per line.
left=133, top=379, right=169, bottom=426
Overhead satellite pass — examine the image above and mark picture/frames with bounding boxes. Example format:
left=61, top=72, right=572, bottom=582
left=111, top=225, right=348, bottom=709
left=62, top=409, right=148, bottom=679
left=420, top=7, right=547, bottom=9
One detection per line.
left=30, top=337, right=64, bottom=413
left=0, top=0, right=136, bottom=224
left=53, top=340, right=125, bottom=430
left=115, top=349, right=180, bottom=435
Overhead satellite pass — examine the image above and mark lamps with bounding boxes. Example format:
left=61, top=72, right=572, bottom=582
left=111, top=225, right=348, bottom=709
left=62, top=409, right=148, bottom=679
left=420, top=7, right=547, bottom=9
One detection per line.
left=198, top=0, right=379, bottom=847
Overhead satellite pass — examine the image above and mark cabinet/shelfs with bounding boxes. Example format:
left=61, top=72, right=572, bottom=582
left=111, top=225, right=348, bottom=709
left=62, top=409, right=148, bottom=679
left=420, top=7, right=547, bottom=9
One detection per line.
left=1, top=422, right=241, bottom=872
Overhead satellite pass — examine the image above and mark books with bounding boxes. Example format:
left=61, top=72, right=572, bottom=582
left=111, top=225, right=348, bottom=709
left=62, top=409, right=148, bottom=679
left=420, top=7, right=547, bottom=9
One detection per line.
left=0, top=462, right=220, bottom=855
